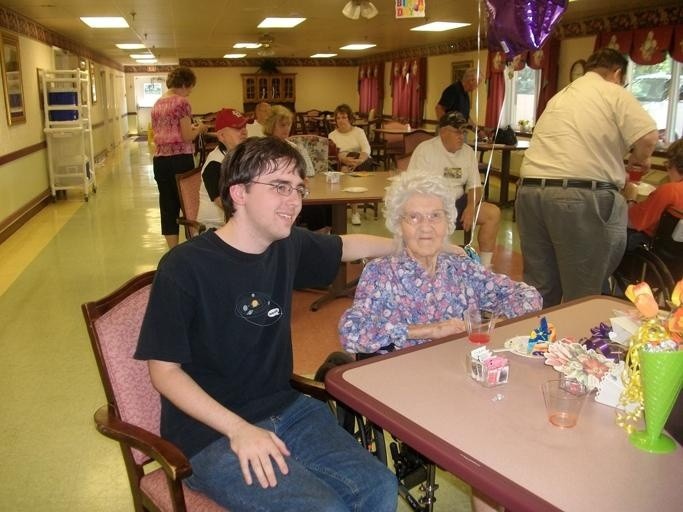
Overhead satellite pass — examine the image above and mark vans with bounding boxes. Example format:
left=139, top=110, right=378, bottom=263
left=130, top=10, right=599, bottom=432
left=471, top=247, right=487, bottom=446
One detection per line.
left=625, top=73, right=683, bottom=143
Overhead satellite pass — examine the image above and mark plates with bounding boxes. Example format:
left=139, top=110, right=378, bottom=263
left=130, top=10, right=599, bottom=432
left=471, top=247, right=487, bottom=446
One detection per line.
left=345, top=187, right=367, bottom=192
left=505, top=336, right=548, bottom=357
left=324, top=172, right=344, bottom=176
left=630, top=183, right=657, bottom=196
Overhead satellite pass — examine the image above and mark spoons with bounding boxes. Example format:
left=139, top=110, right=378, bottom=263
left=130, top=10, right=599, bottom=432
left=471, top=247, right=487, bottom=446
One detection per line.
left=493, top=348, right=528, bottom=354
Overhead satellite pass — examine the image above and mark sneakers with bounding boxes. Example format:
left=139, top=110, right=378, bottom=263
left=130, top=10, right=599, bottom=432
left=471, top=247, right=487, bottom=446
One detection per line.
left=351, top=212, right=361, bottom=225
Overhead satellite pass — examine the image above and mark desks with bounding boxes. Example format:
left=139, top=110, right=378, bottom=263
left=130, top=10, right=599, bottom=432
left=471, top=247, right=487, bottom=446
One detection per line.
left=372, top=128, right=436, bottom=135
left=296, top=170, right=403, bottom=311
left=324, top=294, right=683, bottom=512
left=467, top=140, right=529, bottom=209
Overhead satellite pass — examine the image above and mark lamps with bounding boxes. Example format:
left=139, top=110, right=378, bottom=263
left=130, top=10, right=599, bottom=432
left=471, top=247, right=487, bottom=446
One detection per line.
left=341, top=0, right=361, bottom=20
left=360, top=0, right=379, bottom=19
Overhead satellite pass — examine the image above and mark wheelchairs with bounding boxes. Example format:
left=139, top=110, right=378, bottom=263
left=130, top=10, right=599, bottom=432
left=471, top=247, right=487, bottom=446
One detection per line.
left=314, top=257, right=437, bottom=510
left=609, top=205, right=683, bottom=311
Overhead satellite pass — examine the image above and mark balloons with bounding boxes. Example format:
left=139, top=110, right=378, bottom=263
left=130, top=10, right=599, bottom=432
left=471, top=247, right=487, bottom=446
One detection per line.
left=485, top=1, right=569, bottom=63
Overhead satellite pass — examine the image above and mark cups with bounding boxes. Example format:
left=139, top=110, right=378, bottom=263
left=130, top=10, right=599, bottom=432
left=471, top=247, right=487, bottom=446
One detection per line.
left=542, top=381, right=589, bottom=427
left=192, top=117, right=202, bottom=126
left=630, top=165, right=644, bottom=180
left=463, top=309, right=494, bottom=344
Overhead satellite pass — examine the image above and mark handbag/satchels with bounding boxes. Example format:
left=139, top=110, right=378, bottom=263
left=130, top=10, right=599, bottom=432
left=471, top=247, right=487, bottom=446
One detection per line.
left=495, top=125, right=517, bottom=146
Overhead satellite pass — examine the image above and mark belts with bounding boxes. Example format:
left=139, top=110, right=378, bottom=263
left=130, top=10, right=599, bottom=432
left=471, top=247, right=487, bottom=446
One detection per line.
left=518, top=177, right=619, bottom=189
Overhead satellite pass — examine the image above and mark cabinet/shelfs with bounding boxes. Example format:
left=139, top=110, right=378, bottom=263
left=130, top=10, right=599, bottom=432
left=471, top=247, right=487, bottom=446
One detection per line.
left=6, top=70, right=24, bottom=118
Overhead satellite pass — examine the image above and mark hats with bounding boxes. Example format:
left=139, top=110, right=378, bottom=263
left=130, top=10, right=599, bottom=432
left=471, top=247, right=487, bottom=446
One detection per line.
left=438, top=110, right=473, bottom=130
left=214, top=108, right=254, bottom=132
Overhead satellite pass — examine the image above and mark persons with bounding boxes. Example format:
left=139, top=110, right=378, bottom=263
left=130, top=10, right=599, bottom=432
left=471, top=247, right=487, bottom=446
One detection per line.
left=329, top=104, right=371, bottom=173
left=246, top=101, right=271, bottom=137
left=435, top=68, right=487, bottom=141
left=625, top=138, right=683, bottom=261
left=150, top=66, right=209, bottom=248
left=405, top=111, right=501, bottom=272
left=262, top=105, right=315, bottom=178
left=133, top=136, right=467, bottom=512
left=192, top=107, right=249, bottom=235
left=515, top=47, right=659, bottom=308
left=337, top=169, right=543, bottom=354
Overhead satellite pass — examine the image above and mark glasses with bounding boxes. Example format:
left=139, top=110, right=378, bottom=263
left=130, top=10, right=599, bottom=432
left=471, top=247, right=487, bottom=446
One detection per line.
left=250, top=179, right=309, bottom=200
left=663, top=160, right=673, bottom=167
left=399, top=210, right=449, bottom=224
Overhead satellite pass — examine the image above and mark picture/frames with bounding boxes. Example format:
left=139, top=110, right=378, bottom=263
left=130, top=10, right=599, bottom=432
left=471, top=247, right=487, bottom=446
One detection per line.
left=79, top=56, right=88, bottom=105
left=451, top=60, right=470, bottom=109
left=89, top=60, right=97, bottom=103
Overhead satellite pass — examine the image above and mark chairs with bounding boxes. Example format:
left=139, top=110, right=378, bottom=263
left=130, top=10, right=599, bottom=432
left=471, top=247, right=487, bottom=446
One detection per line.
left=396, top=129, right=434, bottom=171
left=382, top=119, right=411, bottom=170
left=306, top=109, right=321, bottom=127
left=81, top=268, right=334, bottom=512
left=194, top=118, right=218, bottom=168
left=174, top=166, right=206, bottom=241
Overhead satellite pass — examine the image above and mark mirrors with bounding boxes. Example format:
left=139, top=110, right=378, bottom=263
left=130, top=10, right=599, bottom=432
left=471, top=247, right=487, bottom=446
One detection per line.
left=0, top=30, right=28, bottom=126
